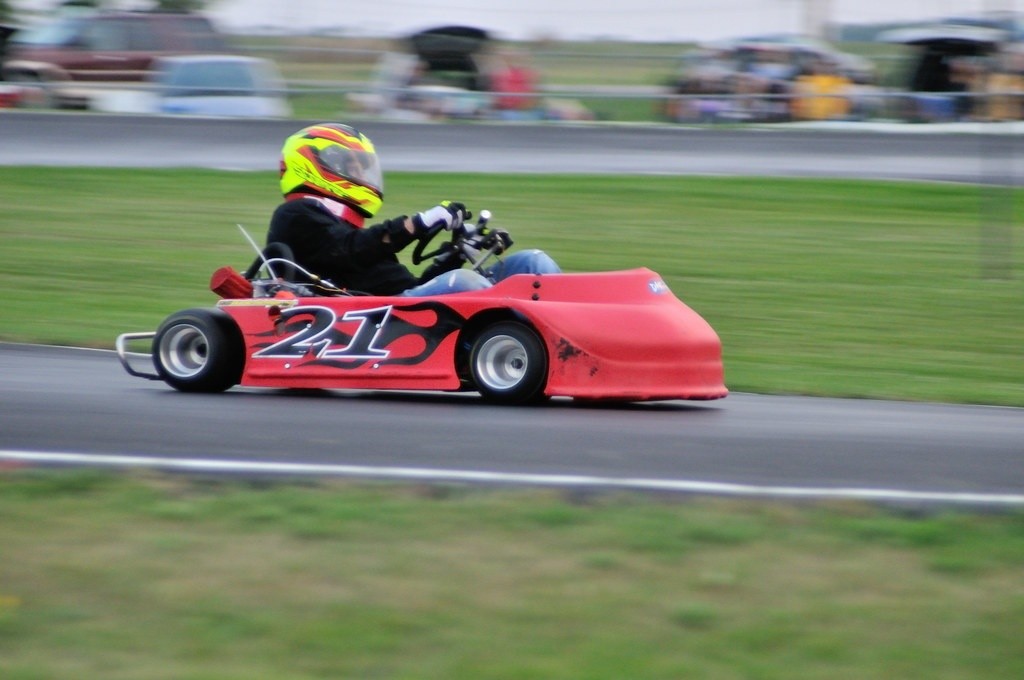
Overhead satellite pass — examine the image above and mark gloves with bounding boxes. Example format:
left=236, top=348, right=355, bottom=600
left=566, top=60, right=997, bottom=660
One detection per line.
left=411, top=200, right=472, bottom=240
left=477, top=224, right=514, bottom=256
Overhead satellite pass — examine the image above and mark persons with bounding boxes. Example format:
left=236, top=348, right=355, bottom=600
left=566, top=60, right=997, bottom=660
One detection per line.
left=372, top=41, right=981, bottom=126
left=264, top=123, right=562, bottom=300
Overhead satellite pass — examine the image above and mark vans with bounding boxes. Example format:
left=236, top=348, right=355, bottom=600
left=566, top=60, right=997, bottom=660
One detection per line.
left=0, top=12, right=224, bottom=83
left=149, top=54, right=289, bottom=118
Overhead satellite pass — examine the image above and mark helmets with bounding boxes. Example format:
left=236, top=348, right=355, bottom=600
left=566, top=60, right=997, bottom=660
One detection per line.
left=279, top=122, right=385, bottom=219
